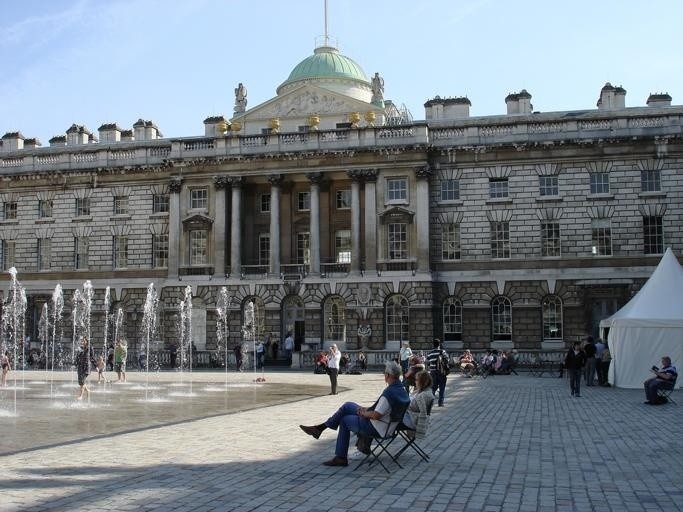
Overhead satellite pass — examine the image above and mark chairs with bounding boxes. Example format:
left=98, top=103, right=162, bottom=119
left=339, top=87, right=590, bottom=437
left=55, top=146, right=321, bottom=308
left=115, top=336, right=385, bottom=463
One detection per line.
left=392, top=399, right=435, bottom=463
left=658, top=383, right=678, bottom=404
left=354, top=402, right=411, bottom=473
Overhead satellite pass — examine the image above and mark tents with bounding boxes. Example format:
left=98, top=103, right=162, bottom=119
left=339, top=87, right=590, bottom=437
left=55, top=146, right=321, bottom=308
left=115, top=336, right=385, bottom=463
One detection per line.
left=600, top=247, right=683, bottom=390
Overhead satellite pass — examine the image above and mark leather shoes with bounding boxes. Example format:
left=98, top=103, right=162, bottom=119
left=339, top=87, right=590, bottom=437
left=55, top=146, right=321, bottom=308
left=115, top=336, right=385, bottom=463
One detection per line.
left=301, top=425, right=321, bottom=440
left=322, top=457, right=347, bottom=467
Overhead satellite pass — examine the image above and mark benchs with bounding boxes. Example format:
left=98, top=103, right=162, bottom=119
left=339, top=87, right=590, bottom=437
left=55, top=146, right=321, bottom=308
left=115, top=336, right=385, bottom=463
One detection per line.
left=317, top=361, right=351, bottom=374
left=520, top=369, right=559, bottom=378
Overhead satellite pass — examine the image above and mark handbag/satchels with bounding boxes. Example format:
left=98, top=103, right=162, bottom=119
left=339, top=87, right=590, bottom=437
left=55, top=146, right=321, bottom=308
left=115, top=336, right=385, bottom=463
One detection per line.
left=438, top=355, right=450, bottom=376
left=601, top=349, right=612, bottom=362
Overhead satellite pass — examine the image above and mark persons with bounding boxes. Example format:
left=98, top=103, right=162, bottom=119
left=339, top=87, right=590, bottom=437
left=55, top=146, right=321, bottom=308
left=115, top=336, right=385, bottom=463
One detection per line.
left=73, top=335, right=90, bottom=399
left=170, top=341, right=196, bottom=368
left=399, top=339, right=519, bottom=408
left=299, top=360, right=410, bottom=465
left=644, top=356, right=677, bottom=405
left=139, top=340, right=147, bottom=370
left=2, top=356, right=9, bottom=385
left=234, top=342, right=242, bottom=371
left=256, top=332, right=294, bottom=365
left=91, top=339, right=126, bottom=382
left=563, top=334, right=611, bottom=396
left=314, top=351, right=367, bottom=375
left=394, top=369, right=433, bottom=441
left=24, top=347, right=47, bottom=369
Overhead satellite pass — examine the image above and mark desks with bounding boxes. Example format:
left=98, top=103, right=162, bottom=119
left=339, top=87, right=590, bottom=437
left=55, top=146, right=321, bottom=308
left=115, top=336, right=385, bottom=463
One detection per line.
left=536, top=360, right=561, bottom=378
